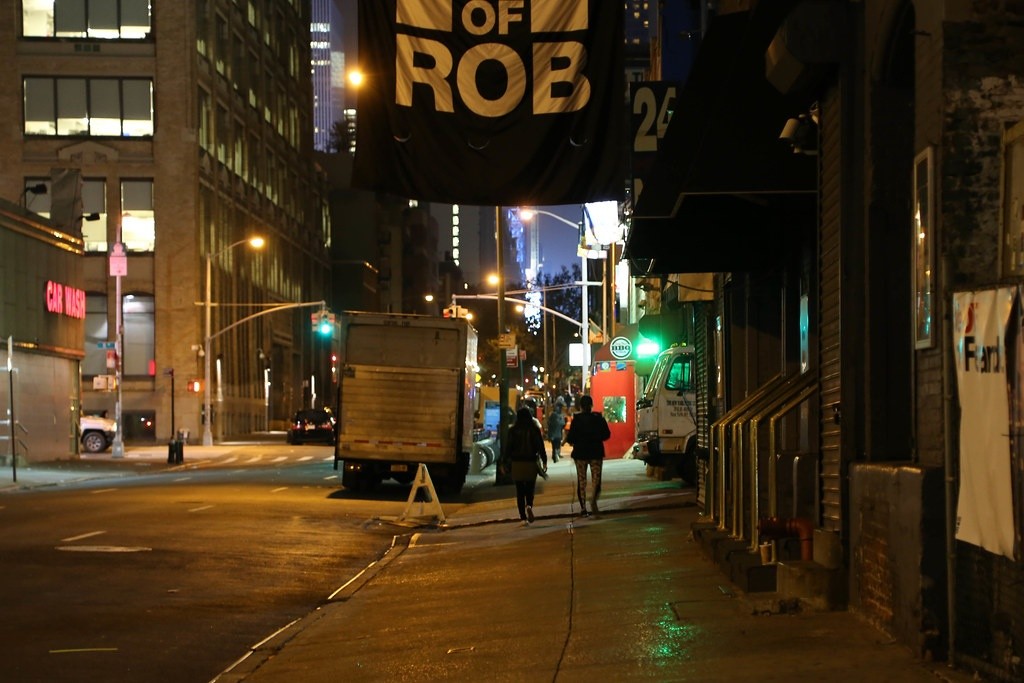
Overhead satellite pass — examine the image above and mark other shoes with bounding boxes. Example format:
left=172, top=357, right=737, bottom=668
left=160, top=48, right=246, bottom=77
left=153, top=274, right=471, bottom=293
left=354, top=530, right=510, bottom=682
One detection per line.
left=582, top=511, right=588, bottom=519
left=521, top=521, right=528, bottom=528
left=592, top=508, right=599, bottom=518
left=527, top=506, right=535, bottom=523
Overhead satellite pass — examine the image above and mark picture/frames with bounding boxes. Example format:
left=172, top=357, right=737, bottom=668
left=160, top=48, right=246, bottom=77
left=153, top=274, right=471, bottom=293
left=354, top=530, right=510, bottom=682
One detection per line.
left=912, top=146, right=935, bottom=351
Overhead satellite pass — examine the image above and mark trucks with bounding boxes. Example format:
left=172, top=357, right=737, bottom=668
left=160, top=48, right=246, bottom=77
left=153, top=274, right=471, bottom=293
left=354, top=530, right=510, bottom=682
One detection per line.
left=631, top=342, right=698, bottom=483
left=334, top=310, right=480, bottom=500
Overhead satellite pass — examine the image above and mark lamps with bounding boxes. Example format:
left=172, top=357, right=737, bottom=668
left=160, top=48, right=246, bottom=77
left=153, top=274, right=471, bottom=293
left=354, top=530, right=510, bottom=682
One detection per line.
left=17, top=183, right=47, bottom=205
left=640, top=282, right=660, bottom=293
left=77, top=213, right=100, bottom=222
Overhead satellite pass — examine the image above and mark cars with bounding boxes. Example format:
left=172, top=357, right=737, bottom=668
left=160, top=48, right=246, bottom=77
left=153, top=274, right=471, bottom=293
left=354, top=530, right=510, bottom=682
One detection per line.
left=77, top=415, right=116, bottom=453
left=286, top=406, right=335, bottom=446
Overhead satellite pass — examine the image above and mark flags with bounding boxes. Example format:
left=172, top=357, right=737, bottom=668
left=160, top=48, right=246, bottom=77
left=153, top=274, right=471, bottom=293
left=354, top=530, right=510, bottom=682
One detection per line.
left=348, top=0, right=627, bottom=206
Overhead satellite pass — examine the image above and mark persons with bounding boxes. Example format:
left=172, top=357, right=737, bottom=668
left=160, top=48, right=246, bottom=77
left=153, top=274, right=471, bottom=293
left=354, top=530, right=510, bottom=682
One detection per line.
left=566, top=395, right=611, bottom=520
left=501, top=407, right=548, bottom=527
left=528, top=402, right=575, bottom=464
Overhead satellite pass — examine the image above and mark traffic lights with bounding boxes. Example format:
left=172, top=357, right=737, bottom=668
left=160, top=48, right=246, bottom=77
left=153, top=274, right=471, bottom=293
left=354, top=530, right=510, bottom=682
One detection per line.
left=328, top=312, right=336, bottom=326
left=442, top=304, right=455, bottom=320
left=319, top=310, right=331, bottom=336
left=311, top=310, right=322, bottom=333
left=457, top=305, right=469, bottom=320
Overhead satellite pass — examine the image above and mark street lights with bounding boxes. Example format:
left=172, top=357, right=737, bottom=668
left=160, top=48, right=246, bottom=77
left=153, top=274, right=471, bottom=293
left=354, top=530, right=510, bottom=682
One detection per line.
left=162, top=366, right=177, bottom=465
left=518, top=208, right=589, bottom=397
left=203, top=236, right=265, bottom=448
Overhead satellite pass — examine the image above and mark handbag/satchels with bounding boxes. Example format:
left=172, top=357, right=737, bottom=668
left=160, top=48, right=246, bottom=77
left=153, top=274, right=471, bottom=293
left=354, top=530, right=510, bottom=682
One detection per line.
left=536, top=454, right=549, bottom=480
